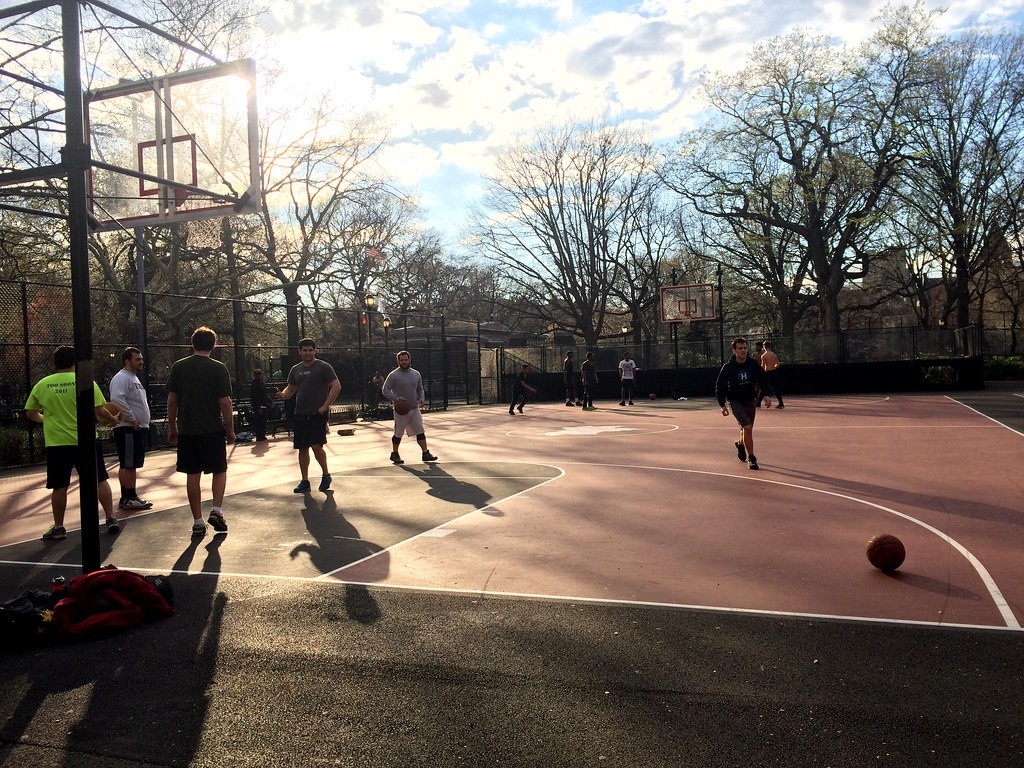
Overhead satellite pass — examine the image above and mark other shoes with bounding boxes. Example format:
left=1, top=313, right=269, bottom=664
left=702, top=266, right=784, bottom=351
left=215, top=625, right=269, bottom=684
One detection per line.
left=629, top=401, right=634, bottom=405
left=582, top=406, right=592, bottom=411
left=566, top=400, right=574, bottom=406
left=576, top=401, right=582, bottom=406
left=619, top=400, right=625, bottom=406
left=517, top=406, right=523, bottom=413
left=258, top=435, right=267, bottom=441
left=509, top=411, right=515, bottom=415
left=588, top=404, right=595, bottom=410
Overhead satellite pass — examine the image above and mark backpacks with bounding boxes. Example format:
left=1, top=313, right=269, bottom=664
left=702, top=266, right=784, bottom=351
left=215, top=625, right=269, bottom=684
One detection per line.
left=0, top=588, right=59, bottom=651
left=236, top=431, right=253, bottom=441
left=51, top=564, right=178, bottom=639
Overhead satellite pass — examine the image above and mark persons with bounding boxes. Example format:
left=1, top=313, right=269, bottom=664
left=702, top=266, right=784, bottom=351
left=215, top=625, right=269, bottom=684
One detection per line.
left=564, top=351, right=583, bottom=406
left=367, top=350, right=437, bottom=463
left=110, top=347, right=152, bottom=509
left=372, top=371, right=384, bottom=397
left=284, top=393, right=296, bottom=413
left=250, top=369, right=269, bottom=441
left=716, top=338, right=771, bottom=470
left=276, top=338, right=342, bottom=493
left=509, top=365, right=536, bottom=415
left=751, top=342, right=785, bottom=409
left=619, top=352, right=636, bottom=406
left=167, top=326, right=236, bottom=535
left=581, top=352, right=599, bottom=411
left=25, top=345, right=121, bottom=539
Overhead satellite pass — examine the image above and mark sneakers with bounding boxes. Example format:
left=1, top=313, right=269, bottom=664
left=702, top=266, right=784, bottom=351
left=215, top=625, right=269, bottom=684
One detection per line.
left=192, top=523, right=207, bottom=535
left=294, top=479, right=311, bottom=493
left=105, top=516, right=119, bottom=529
left=735, top=440, right=746, bottom=462
left=118, top=497, right=153, bottom=510
left=422, top=450, right=437, bottom=461
left=43, top=525, right=68, bottom=539
left=319, top=474, right=331, bottom=491
left=208, top=510, right=228, bottom=531
left=747, top=456, right=759, bottom=470
left=390, top=452, right=404, bottom=464
left=775, top=404, right=785, bottom=409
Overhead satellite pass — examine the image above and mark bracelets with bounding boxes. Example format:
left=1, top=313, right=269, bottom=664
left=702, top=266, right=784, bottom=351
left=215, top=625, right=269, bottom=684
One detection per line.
left=594, top=375, right=597, bottom=378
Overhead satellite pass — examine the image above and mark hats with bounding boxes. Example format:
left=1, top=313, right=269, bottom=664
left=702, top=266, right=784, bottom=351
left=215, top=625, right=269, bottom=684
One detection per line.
left=253, top=369, right=264, bottom=375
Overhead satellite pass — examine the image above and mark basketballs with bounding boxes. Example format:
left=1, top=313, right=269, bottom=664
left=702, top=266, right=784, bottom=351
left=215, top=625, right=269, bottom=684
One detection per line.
left=395, top=400, right=410, bottom=415
left=96, top=402, right=123, bottom=425
left=649, top=394, right=656, bottom=400
left=866, top=533, right=906, bottom=570
